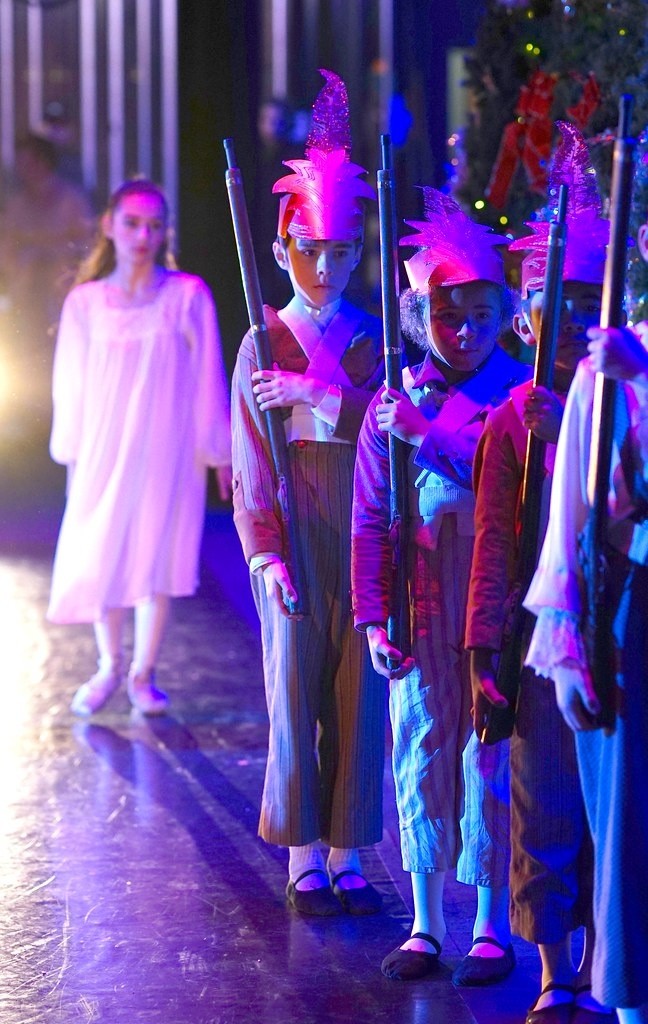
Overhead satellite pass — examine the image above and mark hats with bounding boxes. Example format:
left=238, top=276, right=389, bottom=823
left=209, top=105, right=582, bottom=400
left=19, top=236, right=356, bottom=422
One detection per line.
left=272, top=69, right=377, bottom=242
left=509, top=120, right=609, bottom=300
left=401, top=185, right=511, bottom=294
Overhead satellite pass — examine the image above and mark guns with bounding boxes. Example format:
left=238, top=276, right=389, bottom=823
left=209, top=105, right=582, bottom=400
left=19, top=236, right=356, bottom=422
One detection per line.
left=580, top=92, right=638, bottom=727
left=479, top=183, right=570, bottom=744
left=221, top=135, right=312, bottom=615
left=378, top=130, right=414, bottom=673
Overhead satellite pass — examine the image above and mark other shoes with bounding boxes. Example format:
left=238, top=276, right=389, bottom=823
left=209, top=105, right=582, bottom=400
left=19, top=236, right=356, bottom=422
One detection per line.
left=126, top=660, right=170, bottom=713
left=382, top=933, right=443, bottom=979
left=71, top=657, right=123, bottom=715
left=286, top=869, right=382, bottom=915
left=526, top=983, right=618, bottom=1024
left=451, top=935, right=516, bottom=985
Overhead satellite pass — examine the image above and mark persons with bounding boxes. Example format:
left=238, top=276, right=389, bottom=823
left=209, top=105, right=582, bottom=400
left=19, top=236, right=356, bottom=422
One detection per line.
left=464, top=263, right=626, bottom=1024
left=525, top=223, right=648, bottom=1024
left=230, top=209, right=395, bottom=920
left=351, top=260, right=542, bottom=989
left=3, top=135, right=93, bottom=314
left=49, top=182, right=231, bottom=717
